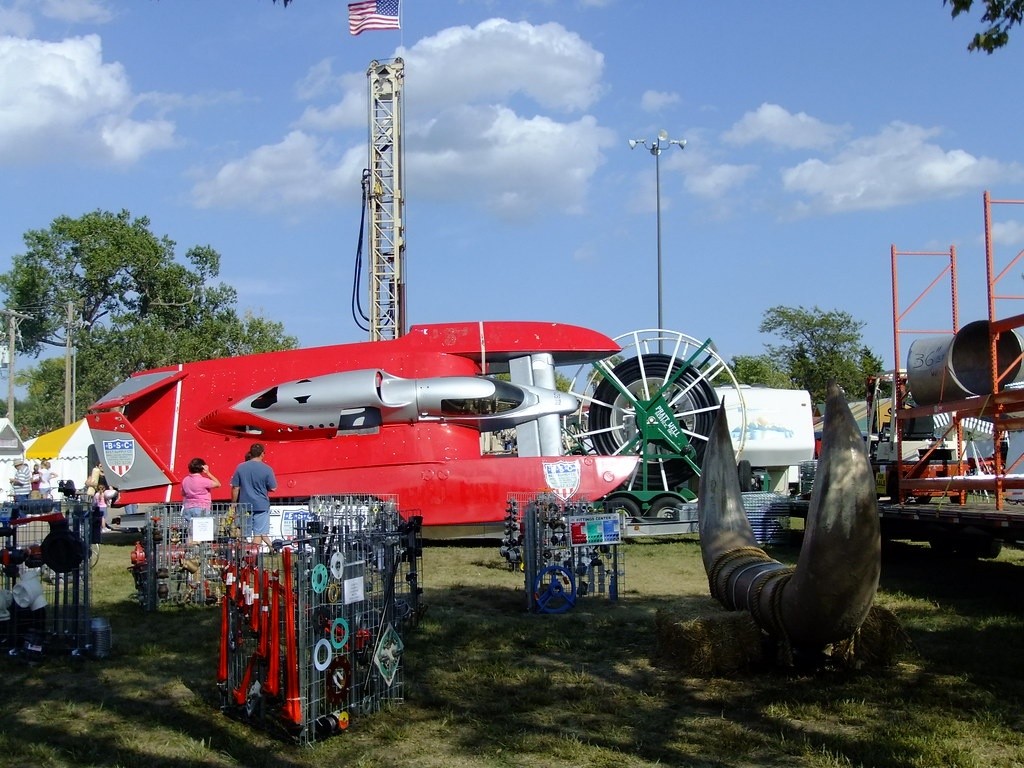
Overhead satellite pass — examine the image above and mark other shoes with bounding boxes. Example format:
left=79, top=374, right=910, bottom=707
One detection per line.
left=102, top=527, right=111, bottom=533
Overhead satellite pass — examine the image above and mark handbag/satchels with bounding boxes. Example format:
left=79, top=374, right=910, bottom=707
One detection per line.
left=221, top=507, right=238, bottom=538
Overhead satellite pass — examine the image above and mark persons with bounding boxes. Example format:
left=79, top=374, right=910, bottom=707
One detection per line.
left=85, top=462, right=137, bottom=532
left=10, top=459, right=58, bottom=501
left=180, top=457, right=220, bottom=545
left=232, top=443, right=279, bottom=557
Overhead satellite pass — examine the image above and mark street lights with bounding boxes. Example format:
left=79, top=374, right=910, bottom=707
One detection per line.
left=627, top=128, right=688, bottom=355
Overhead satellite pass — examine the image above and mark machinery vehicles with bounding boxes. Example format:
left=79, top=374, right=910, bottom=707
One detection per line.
left=861, top=368, right=970, bottom=506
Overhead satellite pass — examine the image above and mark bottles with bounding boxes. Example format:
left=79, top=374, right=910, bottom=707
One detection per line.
left=230, top=519, right=236, bottom=538
